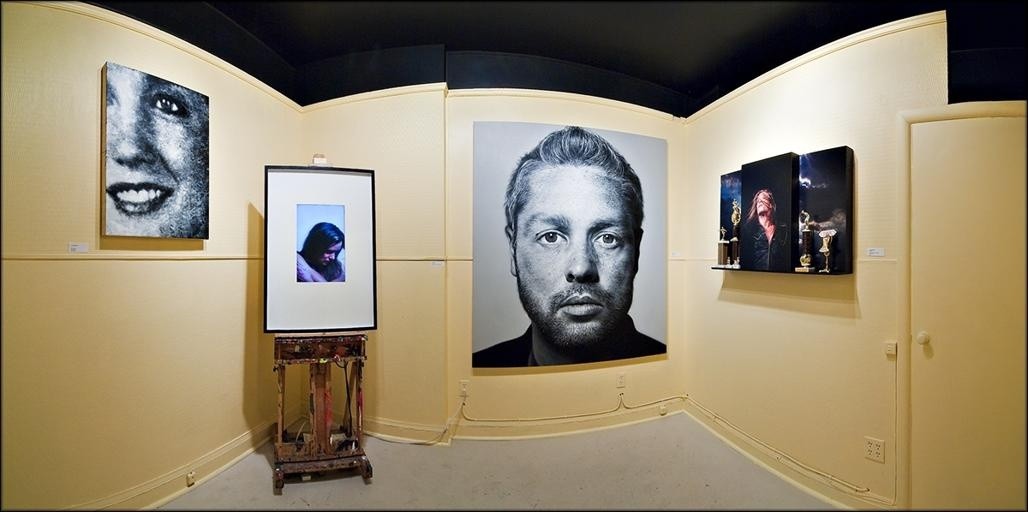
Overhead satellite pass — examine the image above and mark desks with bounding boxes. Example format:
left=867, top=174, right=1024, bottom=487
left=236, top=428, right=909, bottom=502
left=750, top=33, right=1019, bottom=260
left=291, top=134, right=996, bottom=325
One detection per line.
left=269, top=330, right=373, bottom=491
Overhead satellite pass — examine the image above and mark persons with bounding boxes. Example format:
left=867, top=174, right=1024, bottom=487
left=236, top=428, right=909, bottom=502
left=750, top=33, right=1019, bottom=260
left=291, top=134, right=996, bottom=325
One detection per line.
left=740, top=188, right=791, bottom=272
left=469, top=127, right=666, bottom=366
left=721, top=226, right=727, bottom=238
left=105, top=61, right=208, bottom=239
left=729, top=199, right=741, bottom=224
left=298, top=222, right=345, bottom=282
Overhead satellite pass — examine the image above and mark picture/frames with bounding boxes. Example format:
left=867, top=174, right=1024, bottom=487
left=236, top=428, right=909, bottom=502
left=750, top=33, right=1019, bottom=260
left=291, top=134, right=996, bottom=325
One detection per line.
left=264, top=165, right=377, bottom=334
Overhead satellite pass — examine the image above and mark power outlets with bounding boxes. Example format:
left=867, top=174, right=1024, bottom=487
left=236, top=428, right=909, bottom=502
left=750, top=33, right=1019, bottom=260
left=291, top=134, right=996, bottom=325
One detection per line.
left=458, top=379, right=470, bottom=398
left=616, top=372, right=626, bottom=388
left=864, top=436, right=885, bottom=464
